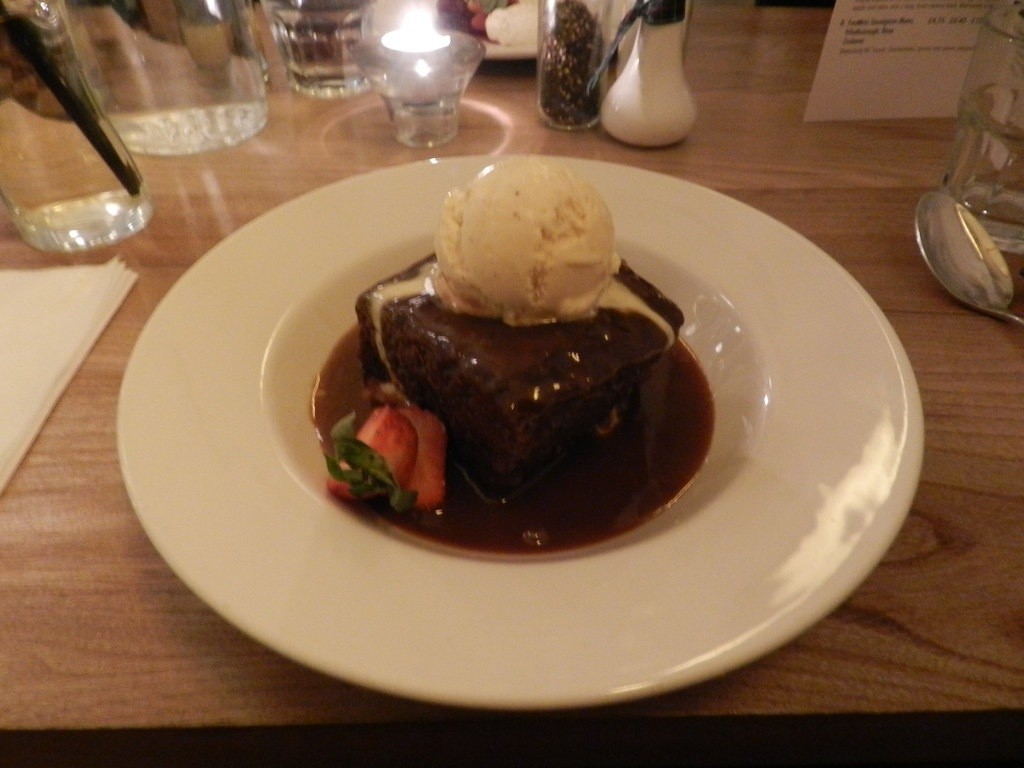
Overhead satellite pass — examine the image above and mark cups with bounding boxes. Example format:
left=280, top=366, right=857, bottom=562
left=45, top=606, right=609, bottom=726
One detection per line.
left=0, top=0, right=153, bottom=251
left=63, top=0, right=270, bottom=154
left=934, top=0, right=1024, bottom=254
left=536, top=0, right=613, bottom=130
left=260, top=0, right=372, bottom=100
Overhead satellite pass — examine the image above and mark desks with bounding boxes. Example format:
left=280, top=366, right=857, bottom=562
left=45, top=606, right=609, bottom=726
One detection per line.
left=0, top=5, right=1024, bottom=767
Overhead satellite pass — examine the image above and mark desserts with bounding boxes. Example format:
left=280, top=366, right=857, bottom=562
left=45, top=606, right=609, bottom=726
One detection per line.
left=353, top=156, right=682, bottom=492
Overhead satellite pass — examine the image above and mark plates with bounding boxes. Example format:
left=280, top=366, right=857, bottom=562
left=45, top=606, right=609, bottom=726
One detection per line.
left=118, top=151, right=925, bottom=711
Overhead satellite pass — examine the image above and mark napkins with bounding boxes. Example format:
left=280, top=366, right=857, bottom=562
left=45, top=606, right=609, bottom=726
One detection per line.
left=0, top=254, right=137, bottom=493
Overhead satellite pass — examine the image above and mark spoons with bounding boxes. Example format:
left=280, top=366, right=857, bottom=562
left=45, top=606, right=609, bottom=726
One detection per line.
left=913, top=191, right=1024, bottom=327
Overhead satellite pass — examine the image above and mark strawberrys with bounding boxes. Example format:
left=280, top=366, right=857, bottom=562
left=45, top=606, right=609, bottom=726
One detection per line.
left=384, top=406, right=447, bottom=509
left=325, top=405, right=418, bottom=501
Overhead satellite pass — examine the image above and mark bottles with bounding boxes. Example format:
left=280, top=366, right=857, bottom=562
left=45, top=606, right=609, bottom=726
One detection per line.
left=600, top=0, right=696, bottom=148
